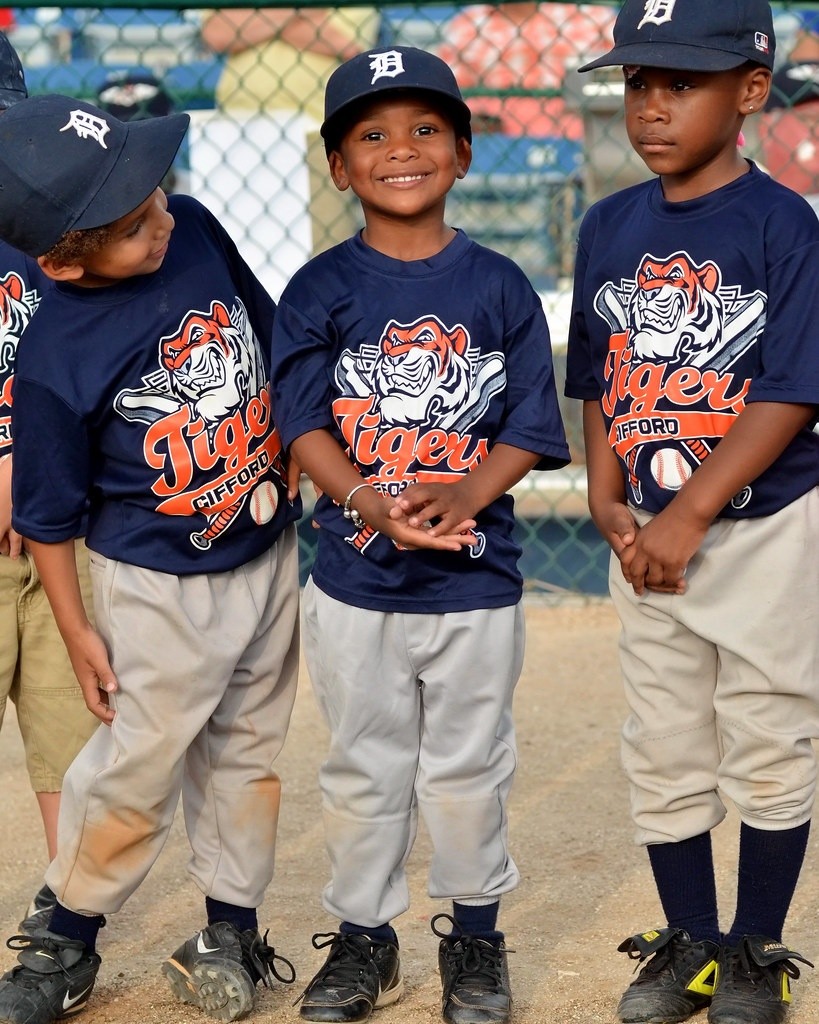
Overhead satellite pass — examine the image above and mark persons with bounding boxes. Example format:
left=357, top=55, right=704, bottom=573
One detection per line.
left=0, top=25, right=97, bottom=861
left=562, top=0, right=819, bottom=1023
left=0, top=95, right=324, bottom=1023
left=198, top=8, right=819, bottom=286
left=271, top=46, right=573, bottom=1024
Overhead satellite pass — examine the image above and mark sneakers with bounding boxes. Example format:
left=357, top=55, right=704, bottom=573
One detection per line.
left=292, top=924, right=405, bottom=1024
left=708, top=930, right=815, bottom=1024
left=160, top=921, right=297, bottom=1022
left=616, top=926, right=725, bottom=1024
left=17, top=892, right=56, bottom=936
left=0, top=928, right=103, bottom=1024
left=429, top=914, right=516, bottom=1024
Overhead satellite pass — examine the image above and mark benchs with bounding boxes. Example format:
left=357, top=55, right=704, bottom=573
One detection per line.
left=1, top=6, right=818, bottom=292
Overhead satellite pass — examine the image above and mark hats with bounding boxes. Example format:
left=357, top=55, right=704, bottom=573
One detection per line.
left=0, top=33, right=27, bottom=96
left=578, top=0, right=776, bottom=72
left=320, top=45, right=469, bottom=159
left=0, top=94, right=189, bottom=258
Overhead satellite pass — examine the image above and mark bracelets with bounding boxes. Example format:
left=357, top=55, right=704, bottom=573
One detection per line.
left=343, top=484, right=378, bottom=528
